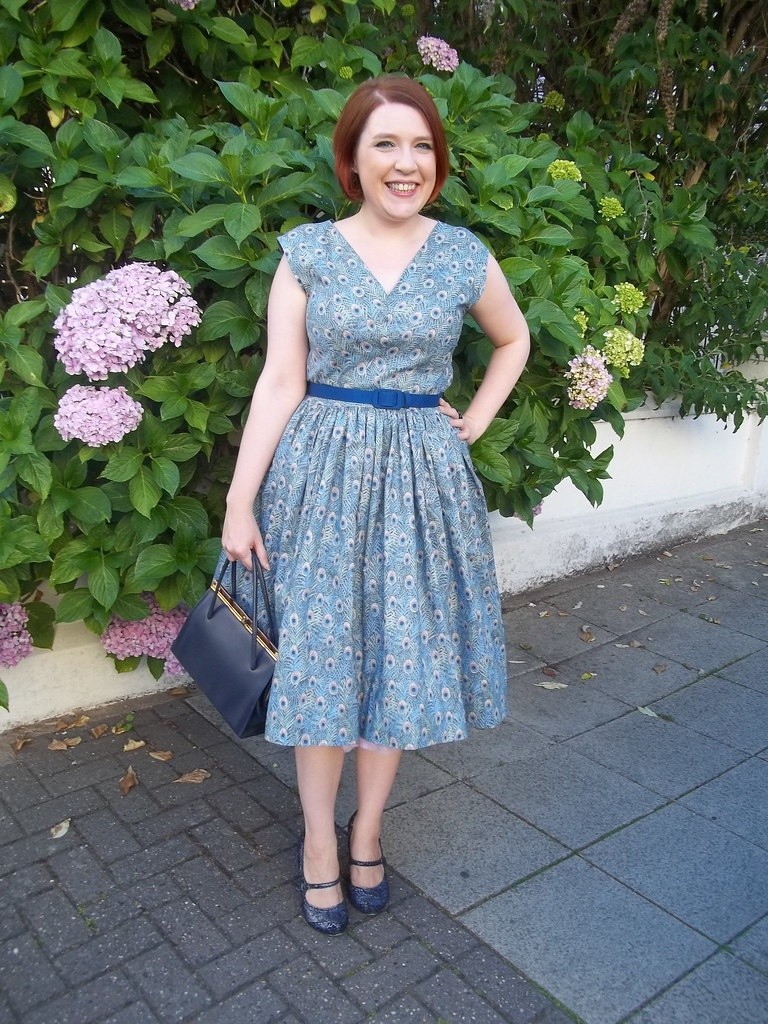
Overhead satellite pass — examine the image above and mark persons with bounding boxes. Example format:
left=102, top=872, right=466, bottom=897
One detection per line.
left=211, top=78, right=532, bottom=932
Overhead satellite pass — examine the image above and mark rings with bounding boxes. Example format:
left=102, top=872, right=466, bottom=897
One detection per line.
left=458, top=412, right=462, bottom=419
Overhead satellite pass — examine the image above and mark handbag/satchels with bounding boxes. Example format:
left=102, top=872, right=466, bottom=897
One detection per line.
left=171, top=549, right=280, bottom=738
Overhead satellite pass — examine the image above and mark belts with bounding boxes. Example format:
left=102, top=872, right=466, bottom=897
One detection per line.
left=305, top=382, right=443, bottom=411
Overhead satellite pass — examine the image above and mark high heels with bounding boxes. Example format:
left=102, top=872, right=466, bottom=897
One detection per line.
left=298, top=830, right=349, bottom=936
left=347, top=809, right=391, bottom=915
